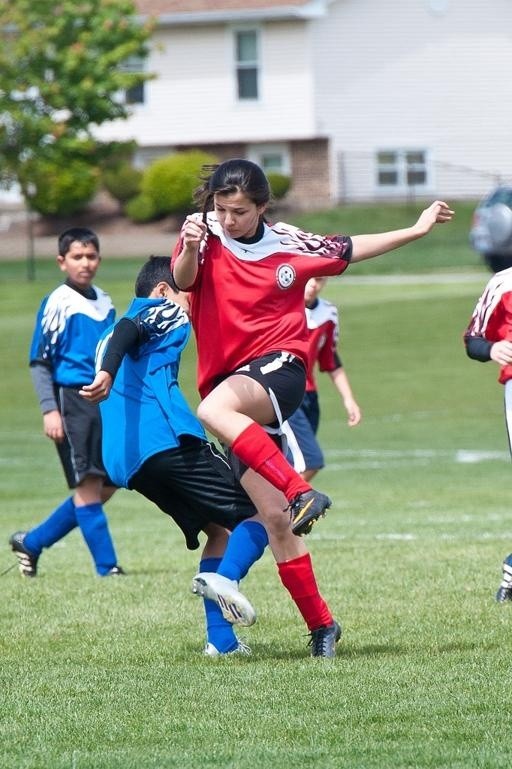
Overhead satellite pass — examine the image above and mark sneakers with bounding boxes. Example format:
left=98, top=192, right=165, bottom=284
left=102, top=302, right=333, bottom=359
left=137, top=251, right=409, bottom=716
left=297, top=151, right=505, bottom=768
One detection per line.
left=205, top=642, right=249, bottom=658
left=190, top=572, right=255, bottom=626
left=308, top=622, right=341, bottom=662
left=10, top=534, right=39, bottom=578
left=291, top=489, right=331, bottom=539
left=497, top=558, right=512, bottom=605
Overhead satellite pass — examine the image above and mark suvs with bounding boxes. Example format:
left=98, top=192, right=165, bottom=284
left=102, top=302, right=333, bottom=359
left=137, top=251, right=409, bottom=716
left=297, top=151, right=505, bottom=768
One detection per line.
left=468, top=184, right=511, bottom=271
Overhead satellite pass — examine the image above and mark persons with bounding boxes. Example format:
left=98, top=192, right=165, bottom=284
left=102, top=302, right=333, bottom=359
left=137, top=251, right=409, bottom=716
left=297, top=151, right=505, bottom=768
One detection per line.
left=9, top=227, right=127, bottom=578
left=170, top=155, right=455, bottom=656
left=282, top=277, right=361, bottom=481
left=79, top=255, right=273, bottom=656
left=463, top=265, right=512, bottom=603
